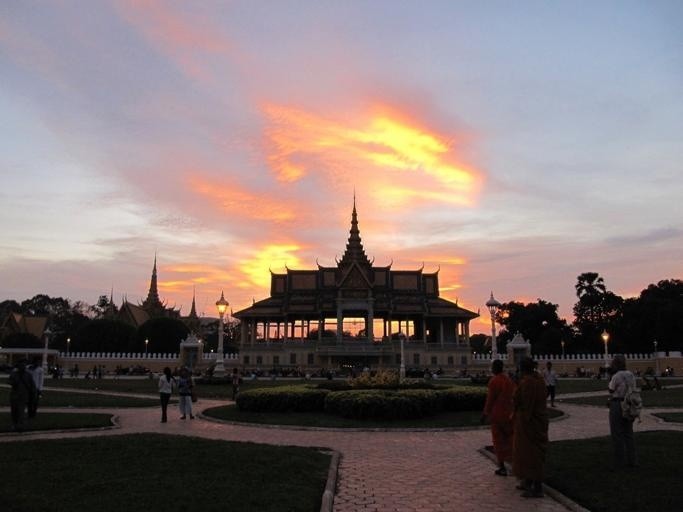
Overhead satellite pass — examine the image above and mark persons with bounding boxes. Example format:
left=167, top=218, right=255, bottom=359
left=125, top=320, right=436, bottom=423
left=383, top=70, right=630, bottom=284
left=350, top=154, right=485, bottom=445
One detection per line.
left=7, top=355, right=45, bottom=431
left=607, top=356, right=642, bottom=471
left=230, top=367, right=241, bottom=401
left=158, top=365, right=195, bottom=423
left=49, top=362, right=121, bottom=385
left=481, top=355, right=560, bottom=497
left=239, top=368, right=333, bottom=380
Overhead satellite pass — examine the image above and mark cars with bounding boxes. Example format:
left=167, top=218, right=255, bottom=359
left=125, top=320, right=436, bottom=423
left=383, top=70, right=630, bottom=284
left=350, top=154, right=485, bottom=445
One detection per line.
left=114, top=365, right=148, bottom=374
left=406, top=364, right=442, bottom=377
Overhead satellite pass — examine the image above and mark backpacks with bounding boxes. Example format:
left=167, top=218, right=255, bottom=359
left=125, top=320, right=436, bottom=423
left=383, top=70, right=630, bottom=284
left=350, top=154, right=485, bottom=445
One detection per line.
left=620, top=377, right=643, bottom=422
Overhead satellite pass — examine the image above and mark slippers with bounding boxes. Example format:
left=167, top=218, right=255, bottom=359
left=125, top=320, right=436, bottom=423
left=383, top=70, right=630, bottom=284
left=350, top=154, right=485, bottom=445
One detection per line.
left=496, top=469, right=544, bottom=499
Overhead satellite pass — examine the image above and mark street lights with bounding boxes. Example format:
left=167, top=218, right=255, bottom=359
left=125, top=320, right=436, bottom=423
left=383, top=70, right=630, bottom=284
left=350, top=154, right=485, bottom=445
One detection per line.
left=560, top=338, right=566, bottom=359
left=144, top=337, right=149, bottom=352
left=399, top=328, right=405, bottom=381
left=215, top=290, right=229, bottom=377
left=485, top=290, right=499, bottom=362
left=42, top=326, right=52, bottom=373
left=653, top=339, right=659, bottom=376
left=600, top=329, right=609, bottom=368
